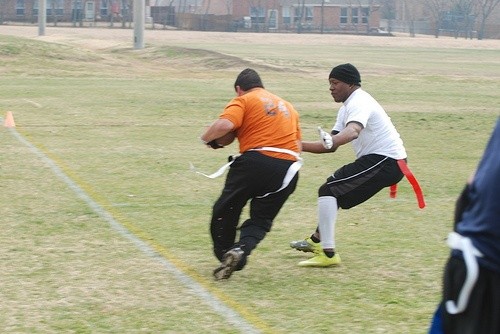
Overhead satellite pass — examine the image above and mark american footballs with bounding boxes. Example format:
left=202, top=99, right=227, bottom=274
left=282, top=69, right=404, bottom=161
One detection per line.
left=217, top=130, right=236, bottom=147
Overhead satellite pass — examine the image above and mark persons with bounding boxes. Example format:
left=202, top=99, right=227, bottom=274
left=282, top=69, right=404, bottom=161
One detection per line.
left=191, top=68, right=302, bottom=282
left=289, top=63, right=425, bottom=267
left=428, top=114, right=500, bottom=334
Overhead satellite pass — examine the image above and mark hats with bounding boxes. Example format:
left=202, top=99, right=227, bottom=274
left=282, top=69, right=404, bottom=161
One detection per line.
left=329, top=63, right=361, bottom=86
left=234, top=68, right=263, bottom=91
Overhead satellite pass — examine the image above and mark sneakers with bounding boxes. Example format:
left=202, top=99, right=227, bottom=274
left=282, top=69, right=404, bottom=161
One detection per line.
left=297, top=250, right=341, bottom=268
left=289, top=236, right=321, bottom=252
left=214, top=248, right=244, bottom=280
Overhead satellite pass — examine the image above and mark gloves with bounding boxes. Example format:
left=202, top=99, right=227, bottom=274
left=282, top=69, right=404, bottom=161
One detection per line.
left=318, top=125, right=333, bottom=149
